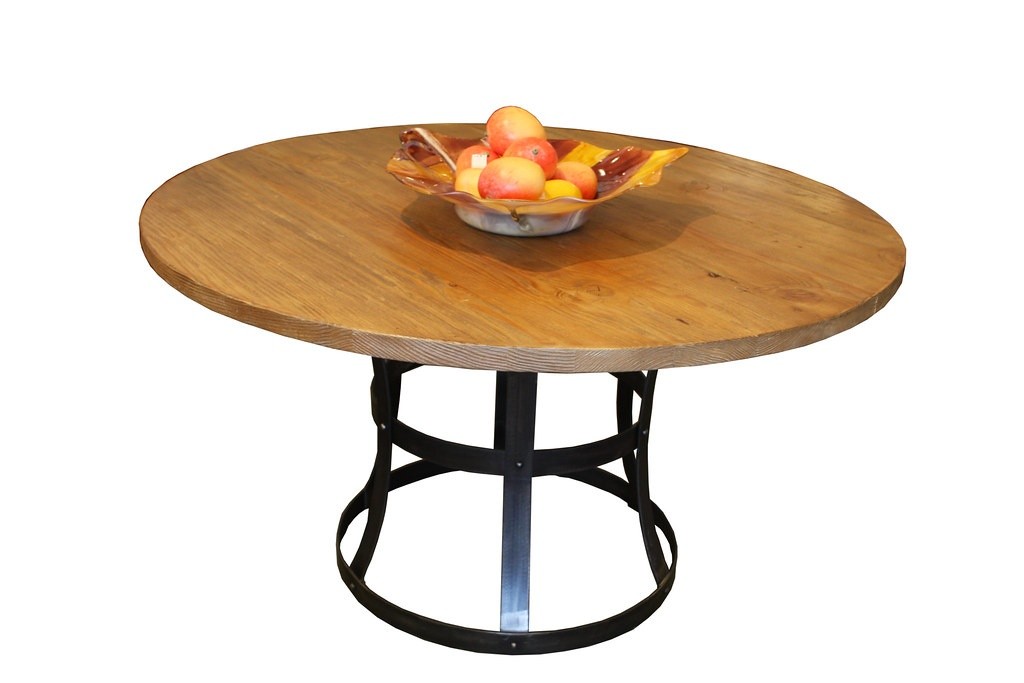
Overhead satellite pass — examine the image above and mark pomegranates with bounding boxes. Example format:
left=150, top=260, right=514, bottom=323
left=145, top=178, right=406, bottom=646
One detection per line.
left=454, top=106, right=597, bottom=200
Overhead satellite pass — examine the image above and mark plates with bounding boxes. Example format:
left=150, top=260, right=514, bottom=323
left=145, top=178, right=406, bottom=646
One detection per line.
left=386, top=128, right=690, bottom=236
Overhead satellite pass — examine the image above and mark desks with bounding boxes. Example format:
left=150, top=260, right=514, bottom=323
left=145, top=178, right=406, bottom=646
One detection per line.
left=135, top=118, right=910, bottom=659
